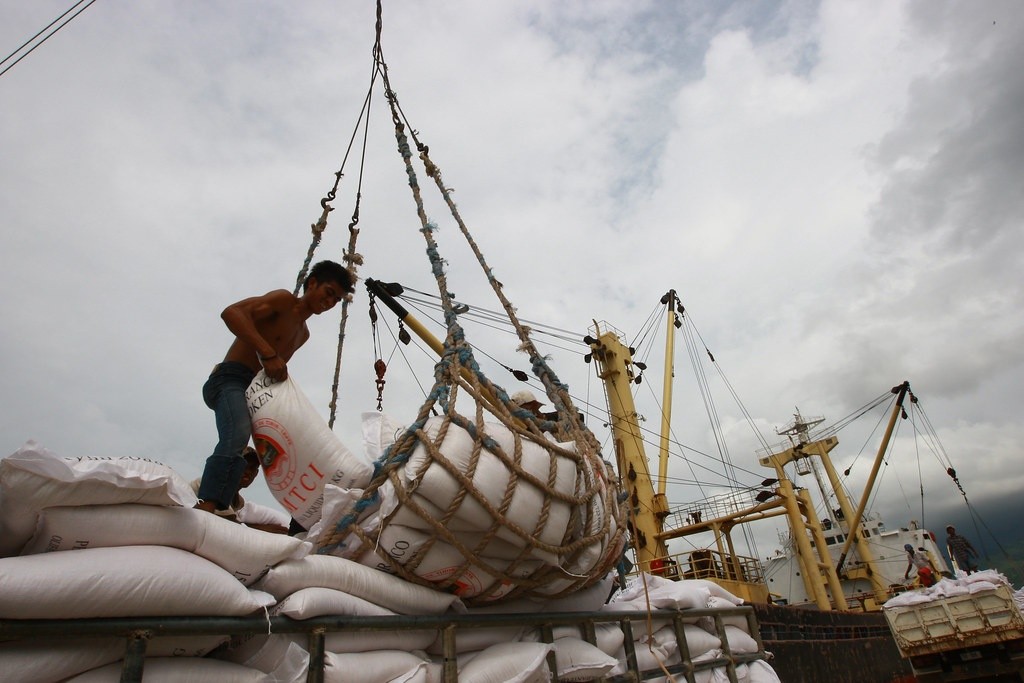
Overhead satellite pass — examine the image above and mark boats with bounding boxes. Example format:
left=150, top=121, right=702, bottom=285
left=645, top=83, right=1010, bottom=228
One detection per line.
left=365, top=273, right=1024, bottom=683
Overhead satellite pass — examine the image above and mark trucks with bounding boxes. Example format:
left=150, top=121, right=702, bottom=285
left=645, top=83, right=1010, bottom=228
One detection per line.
left=881, top=579, right=1023, bottom=683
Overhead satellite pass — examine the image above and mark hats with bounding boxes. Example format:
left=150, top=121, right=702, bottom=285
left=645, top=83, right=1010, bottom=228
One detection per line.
left=511, top=390, right=546, bottom=407
left=904, top=544, right=912, bottom=551
left=243, top=446, right=260, bottom=466
left=946, top=525, right=956, bottom=532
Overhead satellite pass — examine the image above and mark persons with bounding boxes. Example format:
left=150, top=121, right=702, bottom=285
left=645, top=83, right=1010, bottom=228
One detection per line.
left=196, top=261, right=351, bottom=511
left=904, top=544, right=939, bottom=586
left=512, top=390, right=584, bottom=434
left=946, top=525, right=978, bottom=576
left=835, top=508, right=843, bottom=518
left=189, top=445, right=289, bottom=534
